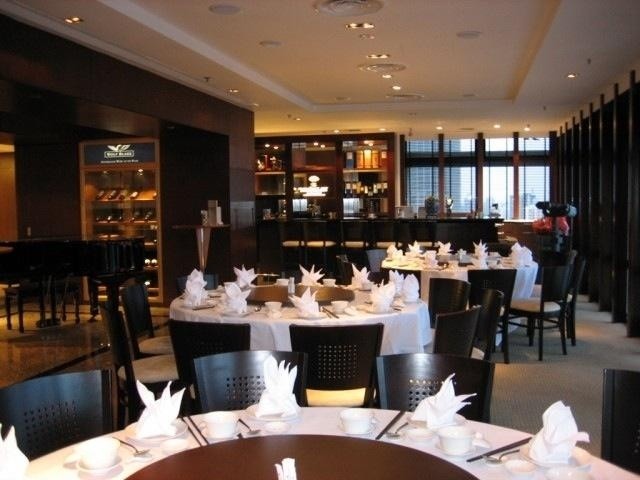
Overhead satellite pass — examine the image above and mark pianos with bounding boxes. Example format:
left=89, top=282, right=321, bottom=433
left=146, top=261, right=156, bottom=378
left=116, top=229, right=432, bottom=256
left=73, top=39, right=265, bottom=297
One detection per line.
left=0, top=236, right=144, bottom=332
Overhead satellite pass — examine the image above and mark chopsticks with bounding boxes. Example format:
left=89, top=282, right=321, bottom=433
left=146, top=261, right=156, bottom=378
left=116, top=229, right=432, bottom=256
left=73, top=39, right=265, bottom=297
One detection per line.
left=376, top=408, right=406, bottom=440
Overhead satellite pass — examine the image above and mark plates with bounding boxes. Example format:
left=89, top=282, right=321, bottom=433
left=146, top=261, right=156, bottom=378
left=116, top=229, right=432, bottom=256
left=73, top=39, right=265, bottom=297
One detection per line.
left=65, top=404, right=302, bottom=474
left=386, top=249, right=532, bottom=269
left=335, top=423, right=376, bottom=435
left=467, top=431, right=594, bottom=480
left=183, top=277, right=420, bottom=320
left=406, top=411, right=491, bottom=455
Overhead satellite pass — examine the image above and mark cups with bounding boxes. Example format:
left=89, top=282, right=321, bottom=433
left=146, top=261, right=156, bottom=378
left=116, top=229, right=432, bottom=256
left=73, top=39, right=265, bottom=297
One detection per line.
left=338, top=409, right=377, bottom=432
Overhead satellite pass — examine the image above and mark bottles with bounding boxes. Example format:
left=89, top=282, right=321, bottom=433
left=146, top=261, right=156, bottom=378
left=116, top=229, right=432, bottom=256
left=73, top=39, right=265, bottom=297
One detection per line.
left=94, top=190, right=157, bottom=223
left=343, top=180, right=387, bottom=216
left=144, top=237, right=158, bottom=289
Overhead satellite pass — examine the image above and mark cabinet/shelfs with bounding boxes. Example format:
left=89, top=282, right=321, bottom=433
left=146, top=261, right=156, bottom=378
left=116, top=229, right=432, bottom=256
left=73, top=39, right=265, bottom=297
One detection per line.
left=81, top=163, right=164, bottom=302
left=255, top=132, right=405, bottom=219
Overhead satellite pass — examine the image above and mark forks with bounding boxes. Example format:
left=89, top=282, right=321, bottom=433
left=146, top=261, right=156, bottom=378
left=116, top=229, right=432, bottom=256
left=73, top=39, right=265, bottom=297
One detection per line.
left=390, top=422, right=408, bottom=437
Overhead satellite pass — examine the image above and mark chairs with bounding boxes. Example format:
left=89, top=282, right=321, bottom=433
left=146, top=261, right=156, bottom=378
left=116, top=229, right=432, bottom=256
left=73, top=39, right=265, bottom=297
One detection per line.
left=276, top=219, right=305, bottom=271
left=339, top=218, right=370, bottom=263
left=371, top=219, right=404, bottom=250
left=302, top=220, right=337, bottom=275
left=408, top=220, right=440, bottom=253
